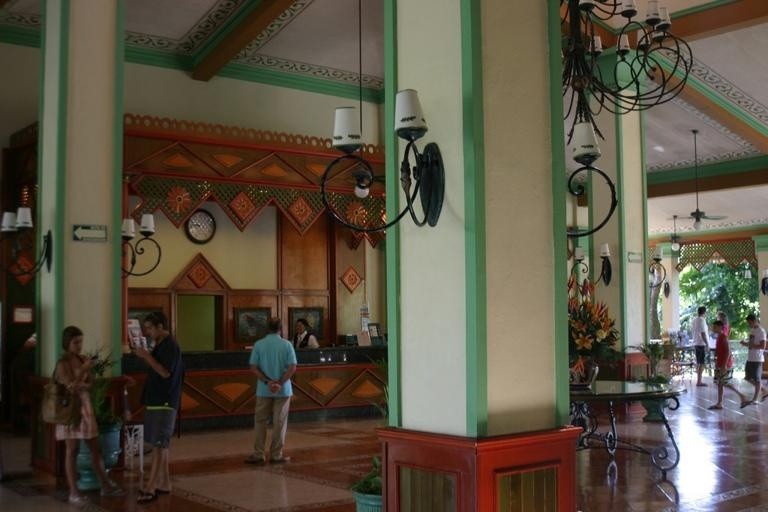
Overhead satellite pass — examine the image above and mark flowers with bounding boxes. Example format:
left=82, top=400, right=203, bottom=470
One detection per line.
left=568, top=277, right=621, bottom=377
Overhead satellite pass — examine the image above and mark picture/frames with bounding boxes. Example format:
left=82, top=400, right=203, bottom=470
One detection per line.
left=233, top=307, right=271, bottom=343
left=127, top=306, right=163, bottom=354
left=288, top=307, right=324, bottom=341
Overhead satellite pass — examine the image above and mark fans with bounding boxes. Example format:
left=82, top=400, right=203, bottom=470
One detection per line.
left=666, top=129, right=728, bottom=221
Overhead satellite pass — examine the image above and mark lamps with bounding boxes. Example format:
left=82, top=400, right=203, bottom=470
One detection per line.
left=671, top=214, right=680, bottom=251
left=567, top=121, right=618, bottom=286
left=648, top=246, right=666, bottom=288
left=561, top=0, right=694, bottom=145
left=353, top=0, right=370, bottom=198
left=121, top=212, right=162, bottom=280
left=0, top=208, right=53, bottom=277
left=320, top=89, right=446, bottom=233
left=693, top=218, right=703, bottom=230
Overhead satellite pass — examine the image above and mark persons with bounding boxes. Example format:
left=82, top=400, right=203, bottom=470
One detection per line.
left=53, top=325, right=128, bottom=507
left=244, top=316, right=298, bottom=465
left=716, top=311, right=730, bottom=339
left=289, top=319, right=321, bottom=348
left=739, top=313, right=767, bottom=405
left=692, top=305, right=711, bottom=387
left=707, top=320, right=752, bottom=410
left=133, top=311, right=186, bottom=506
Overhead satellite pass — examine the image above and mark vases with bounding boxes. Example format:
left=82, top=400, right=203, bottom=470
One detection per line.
left=570, top=353, right=599, bottom=390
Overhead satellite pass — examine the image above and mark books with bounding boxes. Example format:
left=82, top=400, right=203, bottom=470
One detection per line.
left=126, top=319, right=148, bottom=353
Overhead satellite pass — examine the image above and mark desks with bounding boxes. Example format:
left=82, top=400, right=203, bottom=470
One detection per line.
left=570, top=380, right=688, bottom=470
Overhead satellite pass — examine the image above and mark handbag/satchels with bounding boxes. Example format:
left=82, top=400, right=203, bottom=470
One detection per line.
left=42, top=381, right=80, bottom=424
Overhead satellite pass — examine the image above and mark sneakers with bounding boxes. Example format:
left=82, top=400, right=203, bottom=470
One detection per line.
left=270, top=457, right=292, bottom=465
left=243, top=456, right=265, bottom=463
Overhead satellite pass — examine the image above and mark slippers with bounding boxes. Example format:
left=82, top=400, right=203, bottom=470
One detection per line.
left=708, top=405, right=723, bottom=410
left=101, top=488, right=126, bottom=496
left=696, top=383, right=707, bottom=387
left=740, top=393, right=768, bottom=408
left=69, top=495, right=89, bottom=505
left=136, top=487, right=171, bottom=503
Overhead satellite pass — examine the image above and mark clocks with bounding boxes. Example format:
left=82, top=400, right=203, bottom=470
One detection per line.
left=185, top=208, right=217, bottom=244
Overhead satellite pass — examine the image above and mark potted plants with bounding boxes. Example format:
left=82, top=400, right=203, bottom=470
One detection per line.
left=621, top=337, right=680, bottom=422
left=350, top=456, right=382, bottom=512
left=76, top=346, right=122, bottom=492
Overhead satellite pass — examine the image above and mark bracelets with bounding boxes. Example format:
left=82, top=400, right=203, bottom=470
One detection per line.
left=263, top=378, right=270, bottom=386
